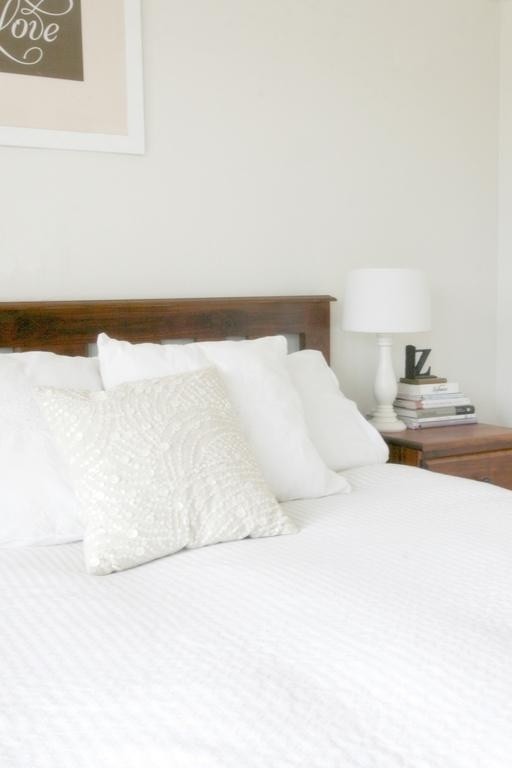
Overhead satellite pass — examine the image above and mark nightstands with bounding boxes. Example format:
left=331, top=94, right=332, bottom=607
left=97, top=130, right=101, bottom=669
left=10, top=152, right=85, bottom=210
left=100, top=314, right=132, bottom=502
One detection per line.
left=380, top=421, right=511, bottom=491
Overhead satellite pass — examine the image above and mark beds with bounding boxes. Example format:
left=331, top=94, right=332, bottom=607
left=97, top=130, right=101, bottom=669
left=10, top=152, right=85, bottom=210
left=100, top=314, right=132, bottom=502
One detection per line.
left=0, top=294, right=511, bottom=768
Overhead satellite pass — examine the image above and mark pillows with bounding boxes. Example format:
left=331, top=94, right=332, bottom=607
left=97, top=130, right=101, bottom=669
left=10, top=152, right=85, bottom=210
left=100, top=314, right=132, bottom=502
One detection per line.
left=0, top=349, right=103, bottom=550
left=286, top=347, right=393, bottom=471
left=40, top=364, right=298, bottom=578
left=97, top=330, right=349, bottom=505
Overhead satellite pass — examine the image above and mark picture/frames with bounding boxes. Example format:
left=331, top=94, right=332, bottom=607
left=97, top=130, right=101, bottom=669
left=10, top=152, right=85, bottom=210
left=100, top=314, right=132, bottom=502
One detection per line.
left=0, top=0, right=149, bottom=156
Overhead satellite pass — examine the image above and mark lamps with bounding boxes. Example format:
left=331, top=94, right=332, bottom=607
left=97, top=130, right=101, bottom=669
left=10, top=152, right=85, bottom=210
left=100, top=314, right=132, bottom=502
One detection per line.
left=345, top=268, right=430, bottom=436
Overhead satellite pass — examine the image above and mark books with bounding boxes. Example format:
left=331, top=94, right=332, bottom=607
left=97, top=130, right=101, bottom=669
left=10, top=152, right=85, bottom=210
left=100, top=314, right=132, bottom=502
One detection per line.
left=364, top=377, right=477, bottom=432
